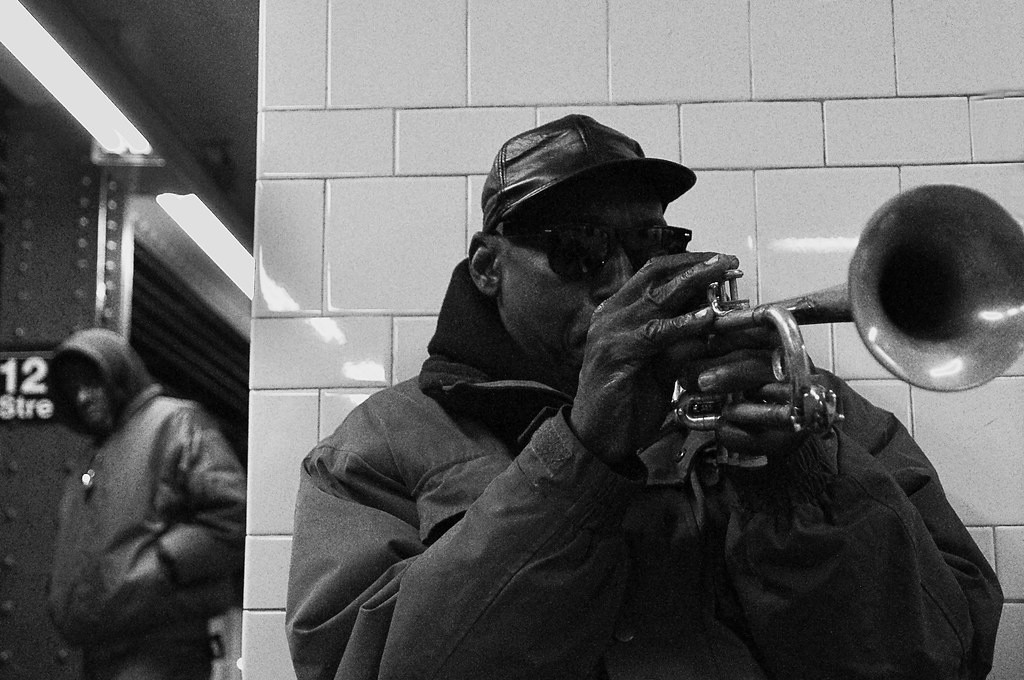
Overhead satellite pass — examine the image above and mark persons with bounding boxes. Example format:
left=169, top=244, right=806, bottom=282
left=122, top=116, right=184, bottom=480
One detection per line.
left=284, top=114, right=1004, bottom=680
left=39, top=328, right=247, bottom=679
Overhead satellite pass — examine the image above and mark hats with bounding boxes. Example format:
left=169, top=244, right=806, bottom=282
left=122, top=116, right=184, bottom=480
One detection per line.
left=482, top=115, right=696, bottom=234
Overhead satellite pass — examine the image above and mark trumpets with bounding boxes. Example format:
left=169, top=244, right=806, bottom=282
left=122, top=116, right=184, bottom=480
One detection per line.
left=664, top=180, right=1024, bottom=457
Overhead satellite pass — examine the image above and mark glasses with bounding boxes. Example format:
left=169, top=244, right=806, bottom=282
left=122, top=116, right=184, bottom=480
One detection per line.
left=490, top=223, right=692, bottom=284
